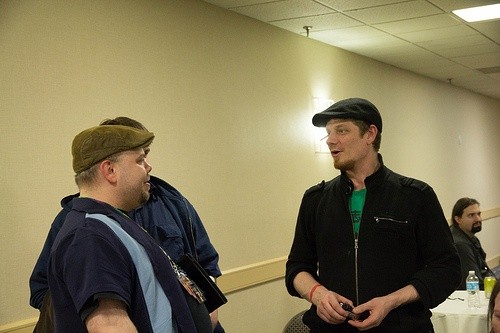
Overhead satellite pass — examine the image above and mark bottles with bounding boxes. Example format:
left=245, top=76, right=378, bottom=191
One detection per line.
left=483, top=272, right=497, bottom=299
left=466, top=270, right=481, bottom=310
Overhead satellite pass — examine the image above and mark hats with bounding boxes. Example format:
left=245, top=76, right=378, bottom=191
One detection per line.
left=312, top=99, right=382, bottom=132
left=71, top=126, right=155, bottom=173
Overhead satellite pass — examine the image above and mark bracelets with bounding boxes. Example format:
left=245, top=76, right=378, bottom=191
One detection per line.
left=308, top=283, right=322, bottom=303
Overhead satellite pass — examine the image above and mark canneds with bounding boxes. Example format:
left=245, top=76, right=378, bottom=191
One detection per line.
left=484, top=276, right=497, bottom=299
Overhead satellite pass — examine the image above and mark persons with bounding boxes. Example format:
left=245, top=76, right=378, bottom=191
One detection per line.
left=447, top=197, right=500, bottom=333
left=29, top=117, right=229, bottom=333
left=285, top=99, right=461, bottom=333
left=45, top=125, right=213, bottom=333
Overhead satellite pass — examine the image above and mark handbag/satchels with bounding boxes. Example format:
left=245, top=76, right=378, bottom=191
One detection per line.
left=182, top=254, right=227, bottom=313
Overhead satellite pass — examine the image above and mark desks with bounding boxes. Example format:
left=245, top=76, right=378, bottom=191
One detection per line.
left=429, top=290, right=492, bottom=333
